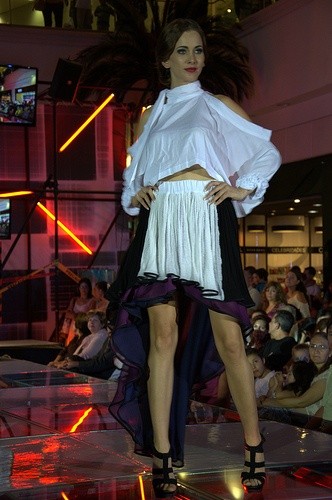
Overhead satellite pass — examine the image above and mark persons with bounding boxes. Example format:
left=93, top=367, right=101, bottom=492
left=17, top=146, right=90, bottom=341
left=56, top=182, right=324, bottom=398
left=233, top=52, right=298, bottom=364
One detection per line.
left=48, top=275, right=122, bottom=381
left=119, top=20, right=282, bottom=497
left=34, top=0, right=272, bottom=34
left=192, top=263, right=332, bottom=421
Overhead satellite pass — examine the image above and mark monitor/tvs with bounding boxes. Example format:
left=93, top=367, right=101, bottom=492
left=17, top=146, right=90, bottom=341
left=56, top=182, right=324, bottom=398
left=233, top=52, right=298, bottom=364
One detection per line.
left=0, top=197, right=13, bottom=240
left=0, top=64, right=37, bottom=126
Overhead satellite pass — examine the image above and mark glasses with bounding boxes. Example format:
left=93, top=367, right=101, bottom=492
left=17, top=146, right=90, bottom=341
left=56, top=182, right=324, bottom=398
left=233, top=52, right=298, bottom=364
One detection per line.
left=309, top=344, right=330, bottom=351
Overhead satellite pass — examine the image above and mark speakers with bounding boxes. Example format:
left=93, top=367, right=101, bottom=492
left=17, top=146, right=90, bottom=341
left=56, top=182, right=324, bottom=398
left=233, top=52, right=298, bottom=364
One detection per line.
left=47, top=58, right=84, bottom=104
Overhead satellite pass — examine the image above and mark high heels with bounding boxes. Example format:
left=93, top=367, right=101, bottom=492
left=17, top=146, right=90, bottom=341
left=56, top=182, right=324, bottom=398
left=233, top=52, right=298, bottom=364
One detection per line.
left=240, top=434, right=266, bottom=491
left=150, top=445, right=177, bottom=498
